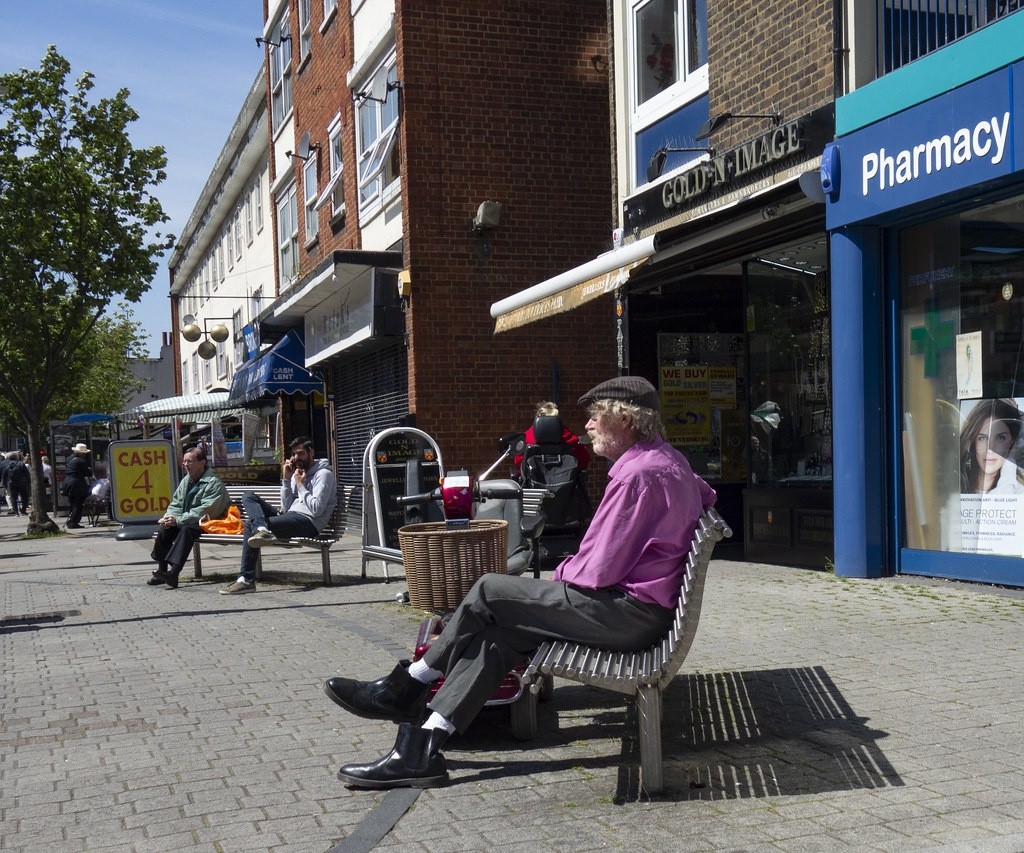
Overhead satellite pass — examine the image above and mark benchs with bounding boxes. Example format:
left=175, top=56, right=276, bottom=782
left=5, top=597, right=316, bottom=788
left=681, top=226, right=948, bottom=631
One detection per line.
left=521, top=506, right=732, bottom=803
left=83, top=486, right=110, bottom=527
left=153, top=486, right=356, bottom=587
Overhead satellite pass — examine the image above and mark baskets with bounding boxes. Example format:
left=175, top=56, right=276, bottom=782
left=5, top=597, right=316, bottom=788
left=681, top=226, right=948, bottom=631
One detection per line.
left=399, top=520, right=508, bottom=609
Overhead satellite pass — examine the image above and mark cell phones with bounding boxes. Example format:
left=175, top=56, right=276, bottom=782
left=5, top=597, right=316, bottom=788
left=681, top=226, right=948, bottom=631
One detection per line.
left=289, top=463, right=297, bottom=472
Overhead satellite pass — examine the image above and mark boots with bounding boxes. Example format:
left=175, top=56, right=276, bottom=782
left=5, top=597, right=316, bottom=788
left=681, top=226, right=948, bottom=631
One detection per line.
left=323, top=660, right=436, bottom=724
left=338, top=724, right=449, bottom=787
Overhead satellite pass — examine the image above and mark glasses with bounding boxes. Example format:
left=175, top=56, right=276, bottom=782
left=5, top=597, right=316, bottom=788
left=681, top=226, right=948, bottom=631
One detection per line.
left=181, top=458, right=204, bottom=464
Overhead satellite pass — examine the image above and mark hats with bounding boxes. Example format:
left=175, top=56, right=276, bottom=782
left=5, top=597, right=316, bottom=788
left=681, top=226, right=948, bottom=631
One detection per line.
left=577, top=377, right=659, bottom=410
left=72, top=443, right=91, bottom=453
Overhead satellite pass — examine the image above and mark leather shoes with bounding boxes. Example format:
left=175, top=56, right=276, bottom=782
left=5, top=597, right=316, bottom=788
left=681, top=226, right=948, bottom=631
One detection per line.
left=147, top=576, right=165, bottom=585
left=152, top=569, right=178, bottom=588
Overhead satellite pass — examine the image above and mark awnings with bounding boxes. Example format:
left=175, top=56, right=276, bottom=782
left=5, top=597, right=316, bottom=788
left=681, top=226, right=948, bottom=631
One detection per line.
left=226, top=327, right=324, bottom=406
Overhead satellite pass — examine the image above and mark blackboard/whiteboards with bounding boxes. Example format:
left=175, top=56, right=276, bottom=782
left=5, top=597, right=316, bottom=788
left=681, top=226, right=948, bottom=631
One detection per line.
left=360, top=426, right=444, bottom=546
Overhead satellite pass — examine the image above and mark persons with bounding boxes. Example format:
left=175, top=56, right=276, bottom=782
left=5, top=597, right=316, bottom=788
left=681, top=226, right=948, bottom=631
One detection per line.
left=514, top=401, right=591, bottom=481
left=63, top=443, right=93, bottom=528
left=324, top=377, right=717, bottom=791
left=960, top=398, right=1024, bottom=495
left=42, top=456, right=52, bottom=484
left=0, top=451, right=31, bottom=517
left=219, top=437, right=337, bottom=594
left=151, top=447, right=231, bottom=588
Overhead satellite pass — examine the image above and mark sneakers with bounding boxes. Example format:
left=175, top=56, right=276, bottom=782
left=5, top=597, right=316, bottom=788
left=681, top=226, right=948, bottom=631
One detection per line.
left=248, top=530, right=277, bottom=547
left=219, top=576, right=256, bottom=594
left=67, top=522, right=85, bottom=529
